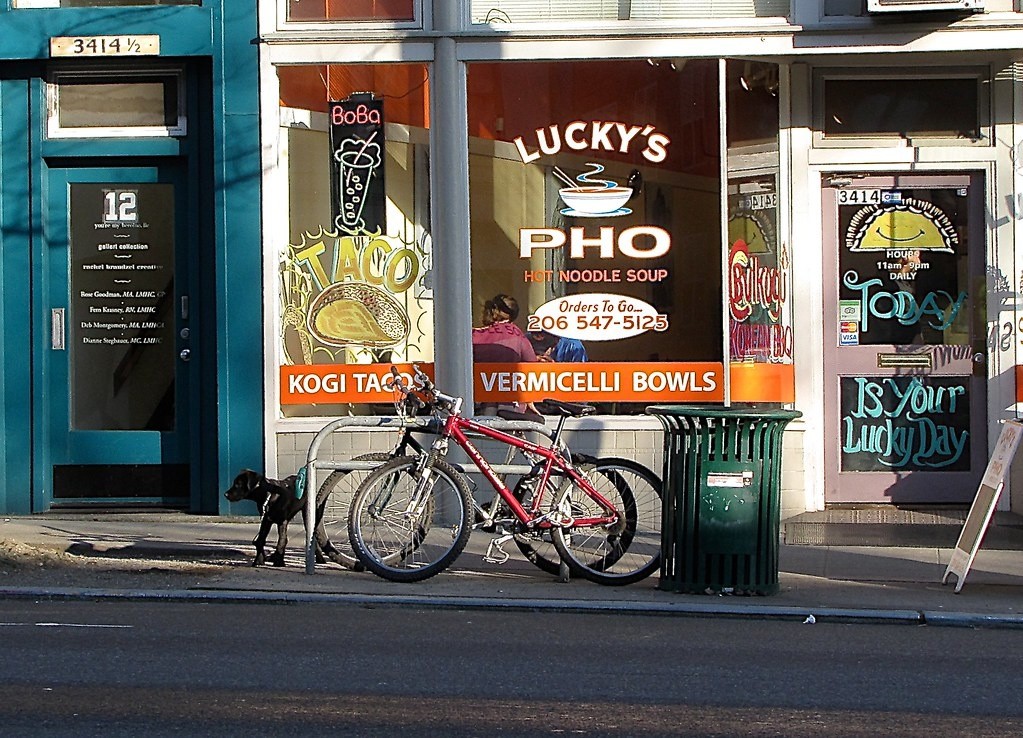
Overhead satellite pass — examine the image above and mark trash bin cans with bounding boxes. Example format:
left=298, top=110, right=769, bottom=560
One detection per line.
left=639, top=405, right=804, bottom=598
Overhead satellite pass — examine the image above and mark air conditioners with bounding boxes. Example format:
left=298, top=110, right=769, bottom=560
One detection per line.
left=867, top=0, right=985, bottom=12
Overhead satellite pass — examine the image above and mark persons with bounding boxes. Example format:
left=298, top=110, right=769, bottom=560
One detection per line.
left=871, top=250, right=932, bottom=470
left=472, top=295, right=588, bottom=415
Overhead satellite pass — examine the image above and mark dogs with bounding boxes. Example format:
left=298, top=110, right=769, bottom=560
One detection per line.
left=223, top=468, right=326, bottom=567
